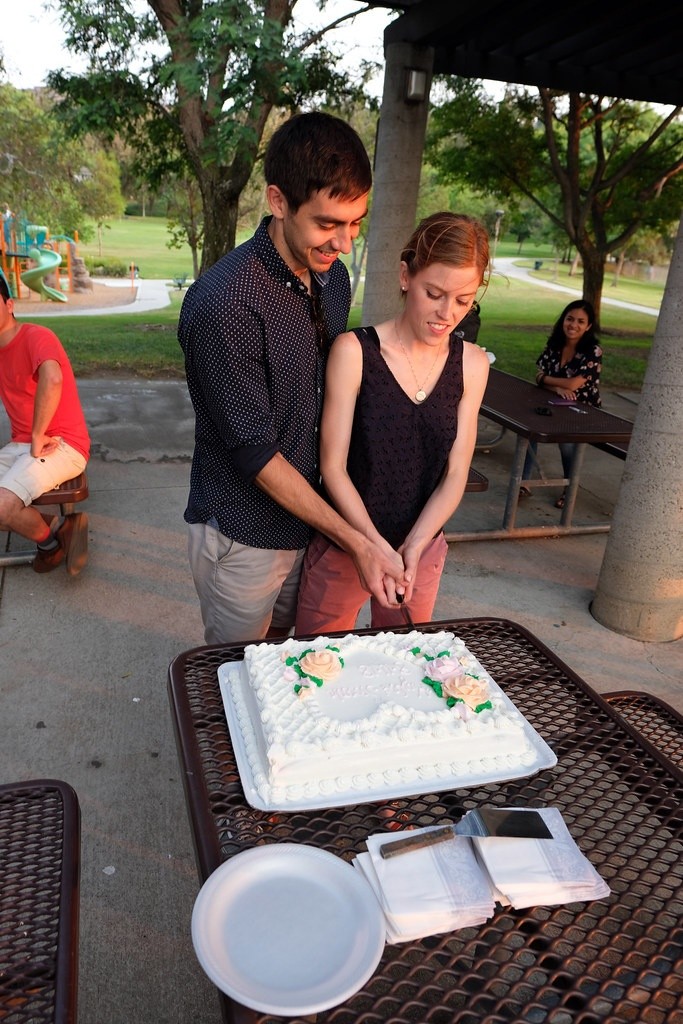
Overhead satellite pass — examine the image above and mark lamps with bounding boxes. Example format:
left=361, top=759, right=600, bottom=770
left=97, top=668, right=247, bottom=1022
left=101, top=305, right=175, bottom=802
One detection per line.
left=403, top=66, right=428, bottom=107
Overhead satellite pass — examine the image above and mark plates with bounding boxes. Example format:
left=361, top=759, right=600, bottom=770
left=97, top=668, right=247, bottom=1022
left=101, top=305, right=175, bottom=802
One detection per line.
left=192, top=842, right=387, bottom=1017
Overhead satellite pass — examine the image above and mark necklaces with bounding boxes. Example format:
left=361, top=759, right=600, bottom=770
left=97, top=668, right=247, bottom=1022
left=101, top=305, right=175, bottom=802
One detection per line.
left=396, top=317, right=442, bottom=401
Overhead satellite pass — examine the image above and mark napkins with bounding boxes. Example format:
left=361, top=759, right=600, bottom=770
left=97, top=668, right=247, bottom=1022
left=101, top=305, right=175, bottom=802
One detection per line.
left=461, top=808, right=612, bottom=910
left=351, top=824, right=496, bottom=945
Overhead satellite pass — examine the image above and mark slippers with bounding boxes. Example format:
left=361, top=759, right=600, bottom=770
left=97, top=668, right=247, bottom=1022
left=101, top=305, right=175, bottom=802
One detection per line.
left=557, top=498, right=565, bottom=508
left=519, top=486, right=535, bottom=500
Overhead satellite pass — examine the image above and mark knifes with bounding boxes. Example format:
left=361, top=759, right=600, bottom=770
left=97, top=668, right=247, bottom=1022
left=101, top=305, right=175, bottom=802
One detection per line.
left=396, top=592, right=415, bottom=632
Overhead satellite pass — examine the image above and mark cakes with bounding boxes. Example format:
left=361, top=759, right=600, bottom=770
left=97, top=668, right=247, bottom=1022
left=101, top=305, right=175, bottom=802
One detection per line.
left=228, top=631, right=538, bottom=802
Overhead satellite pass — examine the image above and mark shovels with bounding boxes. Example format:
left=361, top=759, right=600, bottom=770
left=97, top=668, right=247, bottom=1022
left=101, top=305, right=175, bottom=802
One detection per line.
left=378, top=807, right=555, bottom=859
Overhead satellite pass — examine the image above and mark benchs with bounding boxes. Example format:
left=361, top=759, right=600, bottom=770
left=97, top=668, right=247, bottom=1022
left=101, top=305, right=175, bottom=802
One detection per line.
left=0, top=779, right=82, bottom=1024
left=574, top=690, right=683, bottom=840
left=0, top=472, right=89, bottom=567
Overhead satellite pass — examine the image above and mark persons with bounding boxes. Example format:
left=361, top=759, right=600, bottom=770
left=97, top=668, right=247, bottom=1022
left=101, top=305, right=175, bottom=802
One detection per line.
left=293, top=210, right=489, bottom=632
left=178, top=111, right=408, bottom=645
left=521, top=298, right=603, bottom=508
left=0, top=276, right=91, bottom=574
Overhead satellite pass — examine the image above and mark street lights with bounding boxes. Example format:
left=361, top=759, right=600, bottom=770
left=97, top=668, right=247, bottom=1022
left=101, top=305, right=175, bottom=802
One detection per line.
left=490, top=209, right=505, bottom=266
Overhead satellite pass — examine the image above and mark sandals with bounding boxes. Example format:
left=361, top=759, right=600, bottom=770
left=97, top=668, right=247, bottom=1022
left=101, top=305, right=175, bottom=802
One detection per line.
left=260, top=813, right=281, bottom=832
left=371, top=801, right=413, bottom=831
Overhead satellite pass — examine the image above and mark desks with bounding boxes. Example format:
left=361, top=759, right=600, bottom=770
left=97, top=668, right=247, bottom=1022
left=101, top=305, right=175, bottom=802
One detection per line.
left=166, top=616, right=683, bottom=1024
left=479, top=367, right=635, bottom=531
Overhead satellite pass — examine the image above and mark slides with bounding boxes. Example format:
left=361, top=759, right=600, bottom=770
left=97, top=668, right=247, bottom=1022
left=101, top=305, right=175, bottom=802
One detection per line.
left=19, top=246, right=68, bottom=302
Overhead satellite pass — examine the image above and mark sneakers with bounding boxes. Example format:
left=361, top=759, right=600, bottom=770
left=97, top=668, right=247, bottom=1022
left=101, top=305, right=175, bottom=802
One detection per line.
left=54, top=512, right=89, bottom=577
left=33, top=542, right=65, bottom=573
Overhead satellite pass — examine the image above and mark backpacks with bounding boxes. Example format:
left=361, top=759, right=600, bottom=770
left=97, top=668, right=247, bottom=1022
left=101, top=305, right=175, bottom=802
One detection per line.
left=453, top=300, right=482, bottom=344
